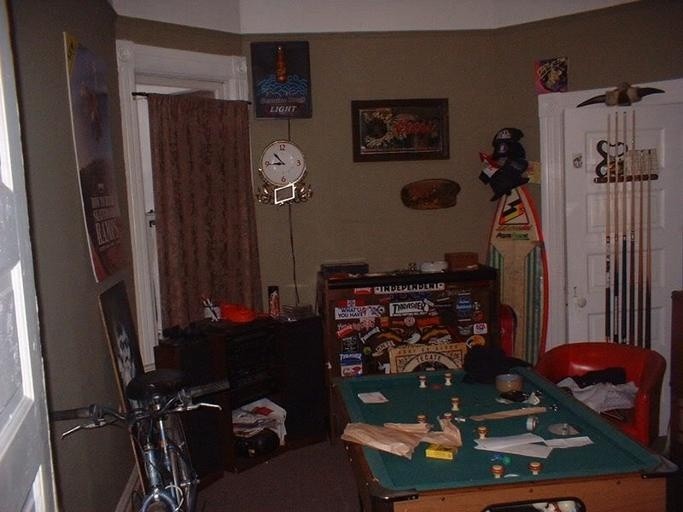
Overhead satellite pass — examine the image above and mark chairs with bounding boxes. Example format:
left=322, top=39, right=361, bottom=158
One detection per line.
left=532, top=341, right=667, bottom=450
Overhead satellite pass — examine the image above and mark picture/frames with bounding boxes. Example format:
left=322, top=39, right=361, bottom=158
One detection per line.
left=352, top=98, right=448, bottom=163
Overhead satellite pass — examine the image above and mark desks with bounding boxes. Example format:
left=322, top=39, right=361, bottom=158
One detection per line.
left=333, top=366, right=678, bottom=512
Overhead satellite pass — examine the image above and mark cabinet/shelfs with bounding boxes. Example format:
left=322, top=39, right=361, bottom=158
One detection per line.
left=153, top=313, right=332, bottom=491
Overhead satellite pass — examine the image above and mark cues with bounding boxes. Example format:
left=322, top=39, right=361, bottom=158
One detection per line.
left=606, top=109, right=653, bottom=350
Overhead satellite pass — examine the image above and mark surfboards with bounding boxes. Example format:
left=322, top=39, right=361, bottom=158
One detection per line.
left=486, top=184, right=549, bottom=371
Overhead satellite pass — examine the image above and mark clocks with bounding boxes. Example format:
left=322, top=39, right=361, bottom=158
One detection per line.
left=255, top=139, right=313, bottom=207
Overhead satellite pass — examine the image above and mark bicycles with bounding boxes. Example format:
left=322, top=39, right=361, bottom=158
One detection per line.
left=51, top=360, right=233, bottom=512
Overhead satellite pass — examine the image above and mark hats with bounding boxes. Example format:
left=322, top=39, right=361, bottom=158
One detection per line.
left=478, top=127, right=531, bottom=202
